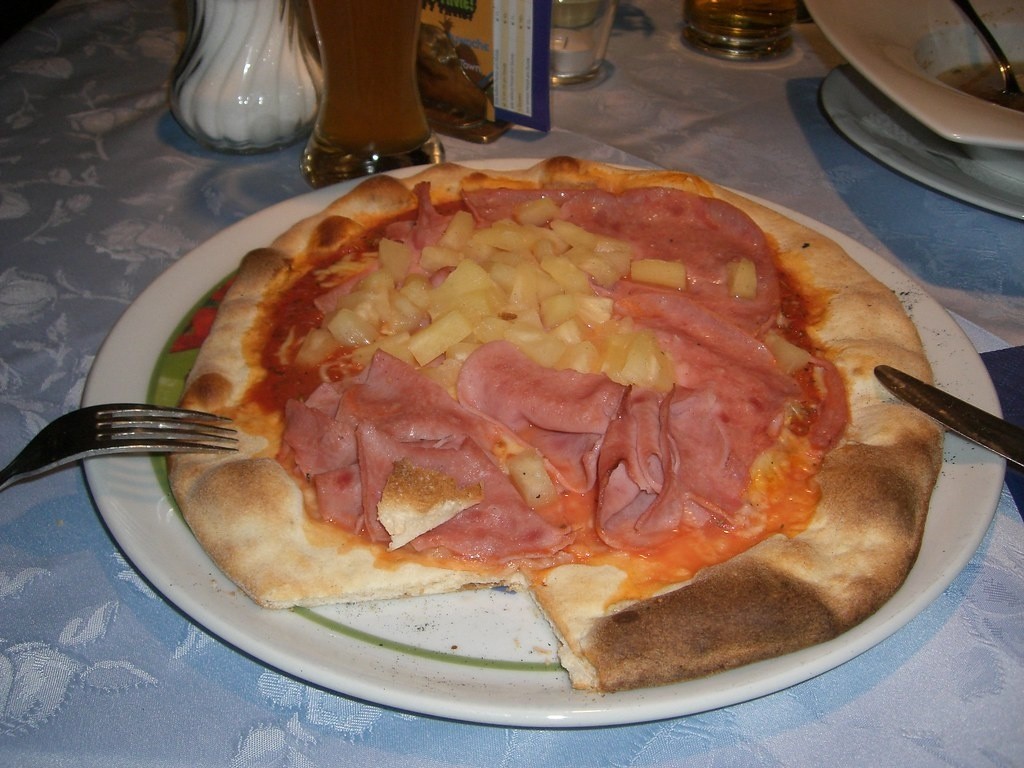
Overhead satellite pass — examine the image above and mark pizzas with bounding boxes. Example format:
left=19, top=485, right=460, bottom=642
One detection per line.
left=168, top=157, right=944, bottom=694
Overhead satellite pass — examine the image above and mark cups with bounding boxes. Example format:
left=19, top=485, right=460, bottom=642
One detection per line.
left=299, top=0, right=445, bottom=190
left=548, top=1, right=619, bottom=82
left=680, top=1, right=795, bottom=62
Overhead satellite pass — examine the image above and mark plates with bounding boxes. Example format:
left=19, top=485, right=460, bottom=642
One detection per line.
left=79, top=161, right=1006, bottom=728
left=821, top=64, right=1024, bottom=222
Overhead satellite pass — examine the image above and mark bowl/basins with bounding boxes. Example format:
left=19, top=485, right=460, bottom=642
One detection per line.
left=807, top=0, right=1023, bottom=150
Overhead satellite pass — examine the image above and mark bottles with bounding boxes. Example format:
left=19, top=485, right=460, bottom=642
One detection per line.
left=168, top=0, right=320, bottom=153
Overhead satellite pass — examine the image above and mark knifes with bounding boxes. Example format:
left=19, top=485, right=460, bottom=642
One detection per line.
left=874, top=366, right=1024, bottom=468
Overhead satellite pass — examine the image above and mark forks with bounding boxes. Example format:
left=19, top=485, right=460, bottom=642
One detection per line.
left=0, top=404, right=238, bottom=491
